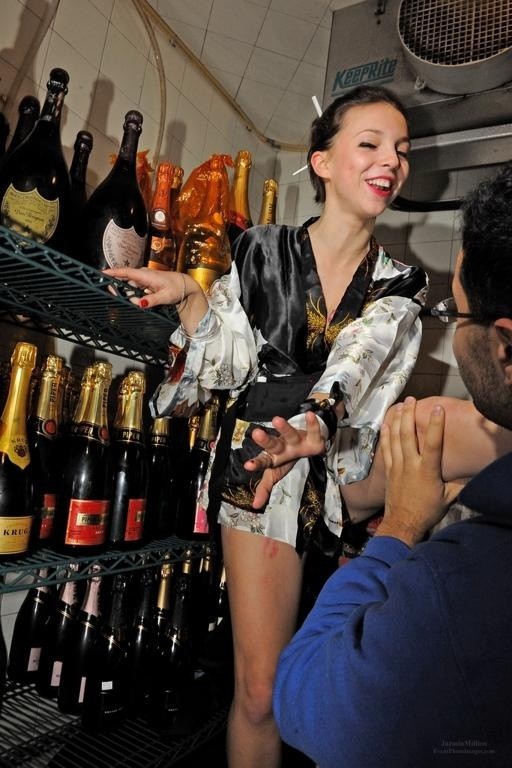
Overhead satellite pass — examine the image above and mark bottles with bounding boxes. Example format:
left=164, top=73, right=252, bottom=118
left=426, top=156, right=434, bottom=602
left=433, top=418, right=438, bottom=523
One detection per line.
left=1, top=68, right=278, bottom=734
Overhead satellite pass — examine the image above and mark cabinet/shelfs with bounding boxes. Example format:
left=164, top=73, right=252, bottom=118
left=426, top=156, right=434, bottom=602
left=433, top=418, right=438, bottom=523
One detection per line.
left=0, top=220, right=223, bottom=768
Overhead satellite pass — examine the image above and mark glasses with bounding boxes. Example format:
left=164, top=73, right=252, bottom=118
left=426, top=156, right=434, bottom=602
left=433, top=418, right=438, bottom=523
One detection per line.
left=430, top=295, right=508, bottom=325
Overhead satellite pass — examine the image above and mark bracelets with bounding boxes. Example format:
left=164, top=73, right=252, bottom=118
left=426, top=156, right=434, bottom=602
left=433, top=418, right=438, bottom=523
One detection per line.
left=178, top=319, right=219, bottom=343
left=298, top=382, right=344, bottom=441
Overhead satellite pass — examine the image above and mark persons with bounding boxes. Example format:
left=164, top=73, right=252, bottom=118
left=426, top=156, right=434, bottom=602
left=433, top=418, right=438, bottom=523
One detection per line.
left=100, top=85, right=512, bottom=768
left=270, top=160, right=512, bottom=768
